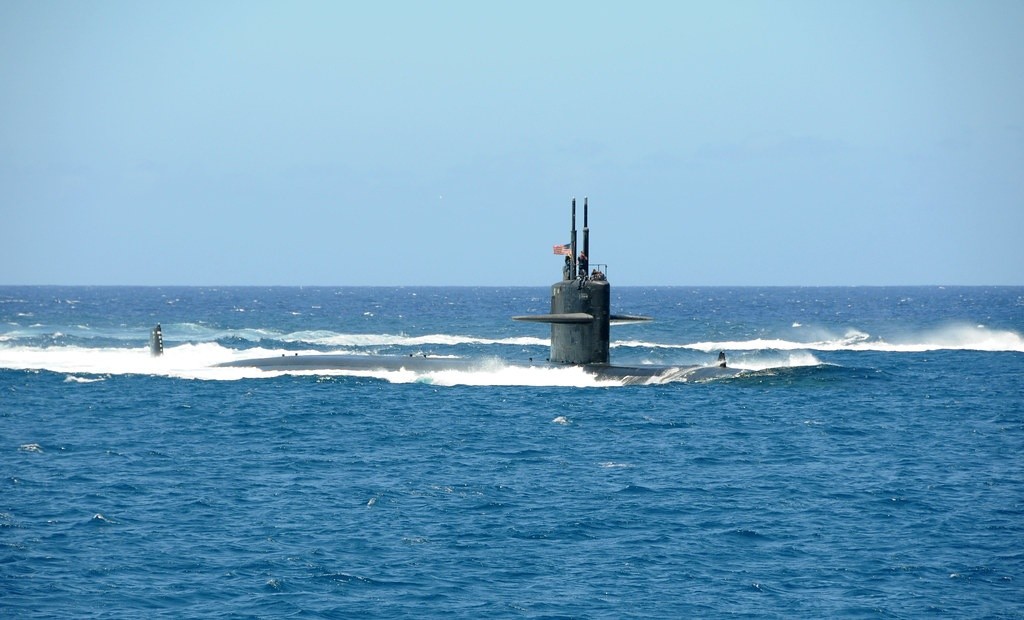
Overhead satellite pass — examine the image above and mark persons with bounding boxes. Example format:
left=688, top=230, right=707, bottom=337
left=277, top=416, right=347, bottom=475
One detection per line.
left=564, top=250, right=607, bottom=280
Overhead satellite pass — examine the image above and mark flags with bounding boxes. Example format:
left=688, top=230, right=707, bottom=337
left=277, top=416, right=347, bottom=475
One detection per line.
left=553, top=242, right=571, bottom=255
